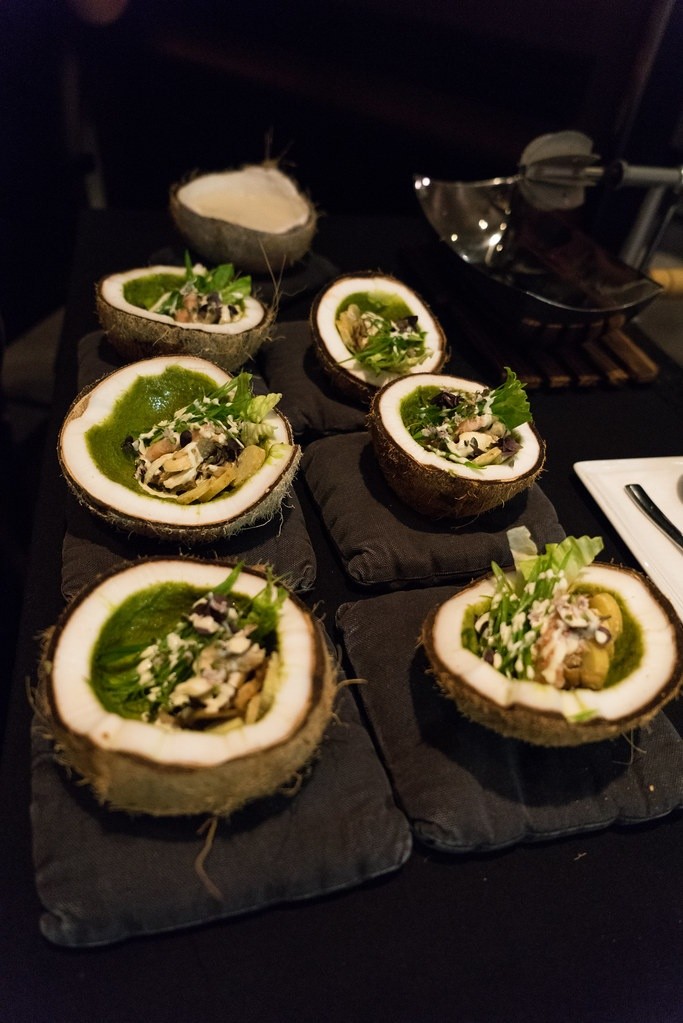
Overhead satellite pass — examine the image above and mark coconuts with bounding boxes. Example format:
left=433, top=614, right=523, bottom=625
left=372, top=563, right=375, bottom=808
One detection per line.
left=56, top=354, right=303, bottom=544
left=171, top=162, right=319, bottom=274
left=309, top=270, right=449, bottom=399
left=94, top=265, right=274, bottom=373
left=419, top=561, right=683, bottom=746
left=40, top=555, right=339, bottom=816
left=368, top=371, right=545, bottom=519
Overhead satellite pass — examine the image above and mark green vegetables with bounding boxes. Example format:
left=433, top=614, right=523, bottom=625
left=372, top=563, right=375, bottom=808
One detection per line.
left=333, top=319, right=431, bottom=378
left=414, top=366, right=534, bottom=467
left=154, top=559, right=292, bottom=687
left=148, top=375, right=283, bottom=450
left=475, top=526, right=604, bottom=671
left=160, top=249, right=254, bottom=315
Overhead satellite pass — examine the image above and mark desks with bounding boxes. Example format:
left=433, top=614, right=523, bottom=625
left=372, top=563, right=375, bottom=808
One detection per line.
left=0, top=190, right=683, bottom=1022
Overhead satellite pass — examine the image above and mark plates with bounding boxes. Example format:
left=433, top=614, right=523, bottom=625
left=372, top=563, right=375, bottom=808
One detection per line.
left=573, top=453, right=682, bottom=624
